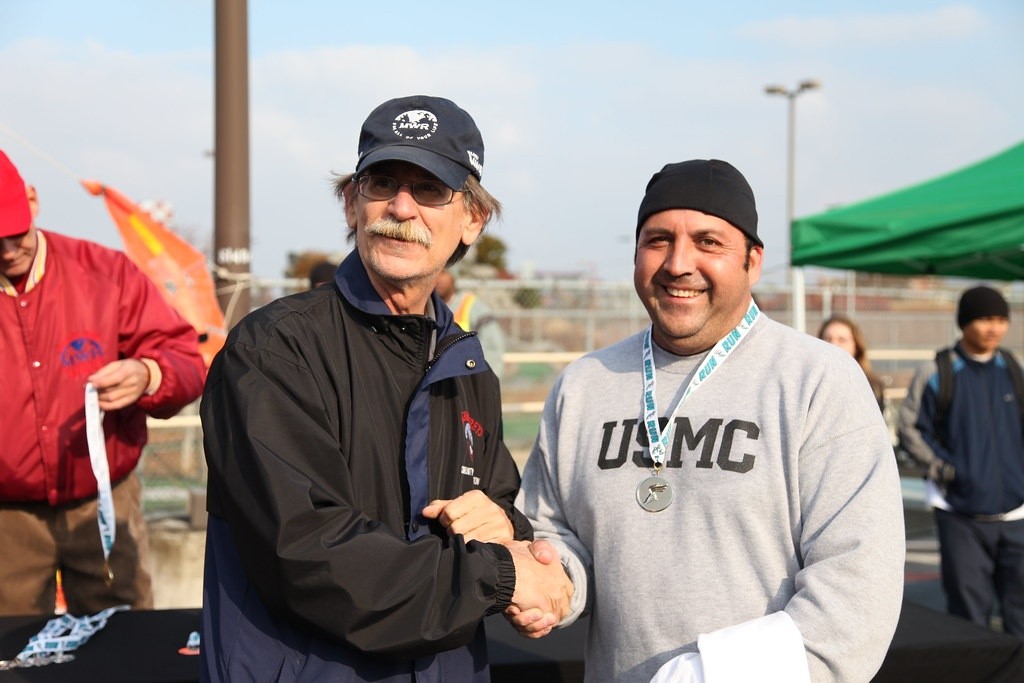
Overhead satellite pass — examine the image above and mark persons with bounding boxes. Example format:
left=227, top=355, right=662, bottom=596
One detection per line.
left=514, top=159, right=906, bottom=683
left=817, top=315, right=901, bottom=450
left=433, top=262, right=502, bottom=378
left=897, top=285, right=1024, bottom=640
left=0, top=148, right=210, bottom=617
left=200, top=95, right=576, bottom=683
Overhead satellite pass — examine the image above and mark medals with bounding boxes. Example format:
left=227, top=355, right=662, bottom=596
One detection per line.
left=101, top=564, right=114, bottom=586
left=178, top=647, right=200, bottom=655
left=636, top=474, right=674, bottom=512
left=1, top=652, right=74, bottom=670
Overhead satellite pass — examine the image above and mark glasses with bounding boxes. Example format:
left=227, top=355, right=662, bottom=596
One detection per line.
left=350, top=173, right=471, bottom=207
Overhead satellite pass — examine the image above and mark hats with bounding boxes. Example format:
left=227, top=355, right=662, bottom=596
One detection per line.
left=0, top=149, right=32, bottom=237
left=956, top=287, right=1009, bottom=331
left=634, top=159, right=763, bottom=247
left=355, top=95, right=484, bottom=191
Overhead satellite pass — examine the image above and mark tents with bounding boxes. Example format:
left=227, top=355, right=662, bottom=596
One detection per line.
left=790, top=140, right=1024, bottom=283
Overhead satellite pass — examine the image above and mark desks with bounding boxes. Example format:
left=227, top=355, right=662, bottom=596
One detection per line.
left=0, top=597, right=1024, bottom=683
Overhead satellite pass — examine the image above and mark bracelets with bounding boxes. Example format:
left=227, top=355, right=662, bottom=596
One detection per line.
left=148, top=360, right=159, bottom=394
left=136, top=358, right=151, bottom=395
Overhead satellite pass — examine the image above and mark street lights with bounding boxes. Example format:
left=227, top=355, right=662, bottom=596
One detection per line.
left=764, top=79, right=818, bottom=332
